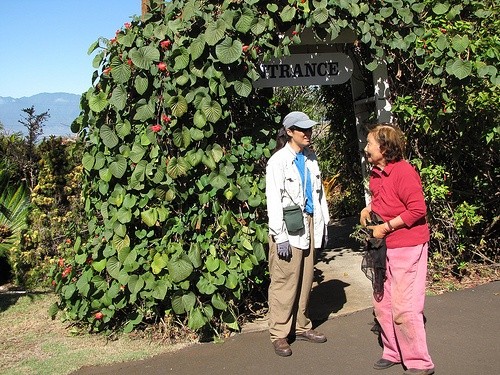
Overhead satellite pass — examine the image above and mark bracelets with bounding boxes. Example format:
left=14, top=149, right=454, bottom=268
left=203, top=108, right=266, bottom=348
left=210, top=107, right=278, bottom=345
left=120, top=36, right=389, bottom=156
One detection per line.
left=381, top=224, right=389, bottom=233
left=387, top=221, right=395, bottom=231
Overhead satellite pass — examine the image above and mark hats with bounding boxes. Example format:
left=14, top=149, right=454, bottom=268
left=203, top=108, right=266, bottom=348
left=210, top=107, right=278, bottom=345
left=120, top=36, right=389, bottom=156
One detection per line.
left=282, top=111, right=318, bottom=129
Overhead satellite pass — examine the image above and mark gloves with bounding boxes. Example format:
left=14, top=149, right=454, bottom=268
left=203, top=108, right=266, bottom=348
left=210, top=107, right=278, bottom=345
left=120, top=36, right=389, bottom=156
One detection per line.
left=277, top=241, right=292, bottom=262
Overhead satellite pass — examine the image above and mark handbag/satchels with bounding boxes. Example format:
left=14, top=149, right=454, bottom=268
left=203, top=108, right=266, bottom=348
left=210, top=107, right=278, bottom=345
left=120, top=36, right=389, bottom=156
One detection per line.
left=283, top=205, right=305, bottom=235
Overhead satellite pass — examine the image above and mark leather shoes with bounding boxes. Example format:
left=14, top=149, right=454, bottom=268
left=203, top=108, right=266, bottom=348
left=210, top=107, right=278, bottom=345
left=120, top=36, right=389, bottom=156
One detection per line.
left=373, top=359, right=394, bottom=370
left=401, top=368, right=435, bottom=375
left=295, top=329, right=326, bottom=343
left=272, top=338, right=292, bottom=357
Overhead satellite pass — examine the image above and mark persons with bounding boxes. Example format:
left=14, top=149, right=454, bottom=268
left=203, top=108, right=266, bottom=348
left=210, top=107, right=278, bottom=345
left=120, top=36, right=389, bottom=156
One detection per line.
left=266, top=111, right=330, bottom=357
left=360, top=123, right=435, bottom=375
